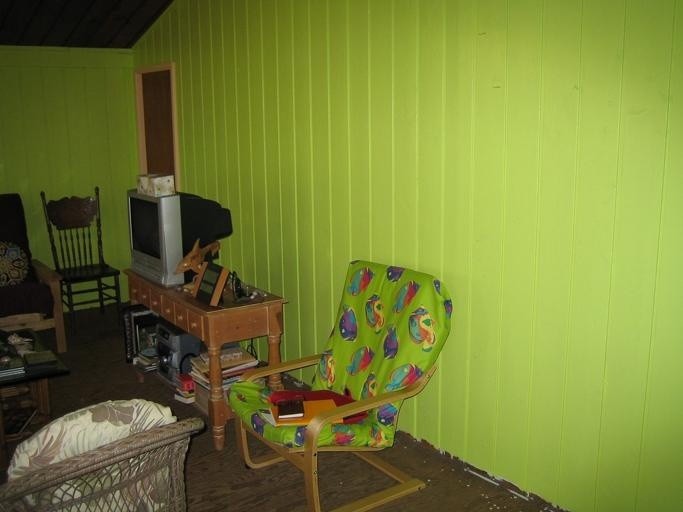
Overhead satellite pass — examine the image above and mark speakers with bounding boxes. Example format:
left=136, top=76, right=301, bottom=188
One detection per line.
left=155, top=322, right=241, bottom=385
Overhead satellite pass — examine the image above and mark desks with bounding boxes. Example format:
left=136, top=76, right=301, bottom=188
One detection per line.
left=122, top=265, right=288, bottom=451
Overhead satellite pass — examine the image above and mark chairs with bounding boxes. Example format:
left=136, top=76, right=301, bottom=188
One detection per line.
left=0, top=193, right=67, bottom=353
left=0, top=417, right=205, bottom=512
left=228, top=260, right=453, bottom=512
left=39, top=186, right=119, bottom=329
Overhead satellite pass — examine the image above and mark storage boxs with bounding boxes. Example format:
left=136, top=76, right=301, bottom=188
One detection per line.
left=194, top=382, right=237, bottom=420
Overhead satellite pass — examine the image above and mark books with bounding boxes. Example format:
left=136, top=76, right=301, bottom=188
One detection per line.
left=120, top=303, right=264, bottom=417
left=0, top=350, right=59, bottom=441
left=270, top=398, right=343, bottom=424
left=257, top=408, right=276, bottom=427
left=276, top=399, right=304, bottom=418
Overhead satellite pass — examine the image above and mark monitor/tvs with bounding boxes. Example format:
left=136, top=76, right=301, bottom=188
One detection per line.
left=127, top=188, right=233, bottom=288
